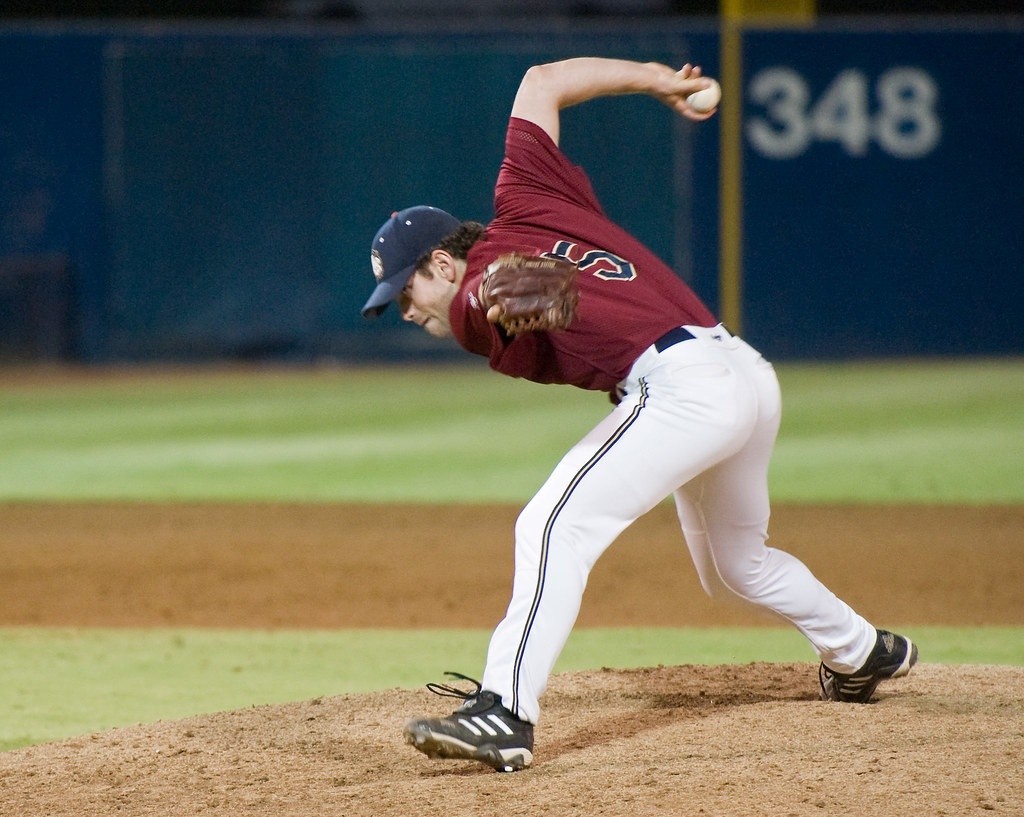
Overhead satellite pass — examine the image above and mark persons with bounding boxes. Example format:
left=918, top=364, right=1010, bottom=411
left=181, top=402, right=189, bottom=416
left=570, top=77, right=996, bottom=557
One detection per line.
left=363, top=55, right=917, bottom=770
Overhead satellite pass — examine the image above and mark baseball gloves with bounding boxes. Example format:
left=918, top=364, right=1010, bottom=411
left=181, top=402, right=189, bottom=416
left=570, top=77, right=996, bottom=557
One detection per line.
left=478, top=251, right=581, bottom=339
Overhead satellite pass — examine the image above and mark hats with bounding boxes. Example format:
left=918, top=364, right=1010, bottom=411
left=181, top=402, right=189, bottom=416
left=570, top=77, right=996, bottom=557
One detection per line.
left=360, top=205, right=461, bottom=318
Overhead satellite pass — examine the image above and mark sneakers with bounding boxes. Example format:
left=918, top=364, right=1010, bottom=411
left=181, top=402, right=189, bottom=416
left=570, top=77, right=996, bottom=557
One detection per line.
left=817, top=630, right=918, bottom=703
left=404, top=672, right=533, bottom=773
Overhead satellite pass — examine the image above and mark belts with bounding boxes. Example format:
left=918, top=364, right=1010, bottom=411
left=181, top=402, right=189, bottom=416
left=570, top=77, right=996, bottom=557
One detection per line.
left=655, top=325, right=721, bottom=353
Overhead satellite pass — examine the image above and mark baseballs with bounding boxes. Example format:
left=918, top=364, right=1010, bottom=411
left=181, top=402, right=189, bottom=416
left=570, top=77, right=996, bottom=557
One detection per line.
left=685, top=77, right=722, bottom=113
